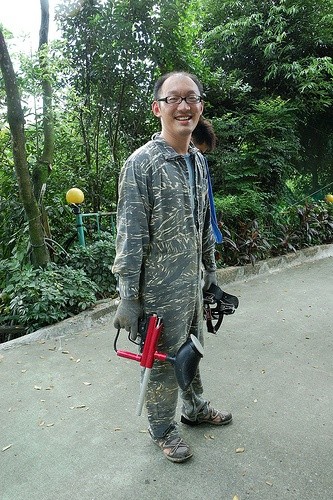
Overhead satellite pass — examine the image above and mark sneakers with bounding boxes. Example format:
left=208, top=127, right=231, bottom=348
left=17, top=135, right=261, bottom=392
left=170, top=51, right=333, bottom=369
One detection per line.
left=180, top=400, right=232, bottom=427
left=148, top=423, right=195, bottom=463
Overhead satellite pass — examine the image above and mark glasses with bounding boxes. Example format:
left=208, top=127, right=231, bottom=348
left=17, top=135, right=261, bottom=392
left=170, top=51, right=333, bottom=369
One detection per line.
left=157, top=95, right=204, bottom=105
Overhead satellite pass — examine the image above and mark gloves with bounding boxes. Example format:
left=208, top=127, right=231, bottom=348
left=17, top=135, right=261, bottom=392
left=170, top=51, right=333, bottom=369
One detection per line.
left=112, top=299, right=143, bottom=341
left=203, top=270, right=218, bottom=291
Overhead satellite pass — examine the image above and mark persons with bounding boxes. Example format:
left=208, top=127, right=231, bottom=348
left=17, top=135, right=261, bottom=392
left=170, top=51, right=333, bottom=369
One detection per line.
left=111, top=72, right=232, bottom=462
